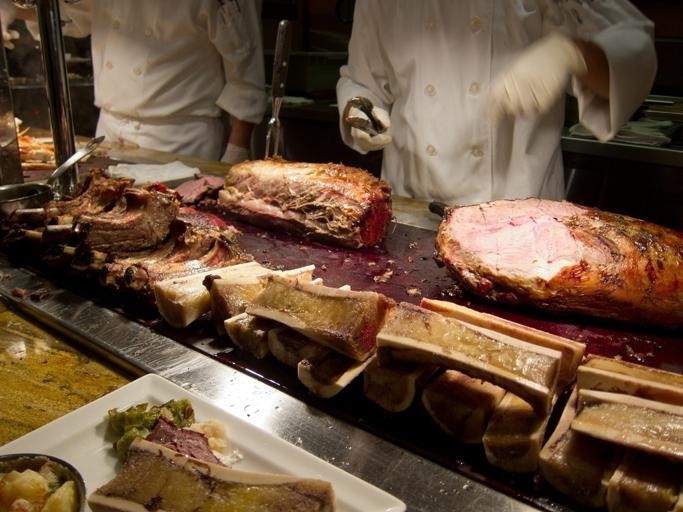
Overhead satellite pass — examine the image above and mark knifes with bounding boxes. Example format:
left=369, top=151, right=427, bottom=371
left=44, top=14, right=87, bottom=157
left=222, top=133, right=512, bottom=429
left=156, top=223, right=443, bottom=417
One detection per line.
left=428, top=201, right=450, bottom=220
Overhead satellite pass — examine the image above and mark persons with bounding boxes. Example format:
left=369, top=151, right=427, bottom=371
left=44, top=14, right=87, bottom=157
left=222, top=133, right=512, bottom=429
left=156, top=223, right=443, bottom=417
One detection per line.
left=8, top=1, right=269, bottom=167
left=336, top=0, right=658, bottom=211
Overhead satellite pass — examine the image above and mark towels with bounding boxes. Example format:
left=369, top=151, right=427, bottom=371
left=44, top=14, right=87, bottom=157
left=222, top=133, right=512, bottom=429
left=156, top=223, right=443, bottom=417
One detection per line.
left=108, top=160, right=201, bottom=186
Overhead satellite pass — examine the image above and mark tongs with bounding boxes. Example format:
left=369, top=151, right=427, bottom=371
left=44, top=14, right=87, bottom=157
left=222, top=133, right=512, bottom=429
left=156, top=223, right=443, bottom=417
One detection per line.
left=343, top=92, right=385, bottom=138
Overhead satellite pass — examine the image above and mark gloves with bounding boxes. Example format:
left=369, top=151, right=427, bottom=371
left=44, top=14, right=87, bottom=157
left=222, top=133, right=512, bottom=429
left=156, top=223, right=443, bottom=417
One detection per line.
left=348, top=104, right=392, bottom=153
left=220, top=143, right=250, bottom=164
left=0, top=0, right=20, bottom=50
left=493, top=32, right=586, bottom=117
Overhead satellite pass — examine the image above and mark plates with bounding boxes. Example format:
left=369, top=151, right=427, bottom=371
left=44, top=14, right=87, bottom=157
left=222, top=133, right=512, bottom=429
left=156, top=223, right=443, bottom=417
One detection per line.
left=0, top=371, right=407, bottom=511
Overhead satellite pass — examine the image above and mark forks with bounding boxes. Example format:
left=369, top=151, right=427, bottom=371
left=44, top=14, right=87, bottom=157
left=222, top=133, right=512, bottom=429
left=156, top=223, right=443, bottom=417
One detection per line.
left=263, top=18, right=293, bottom=157
